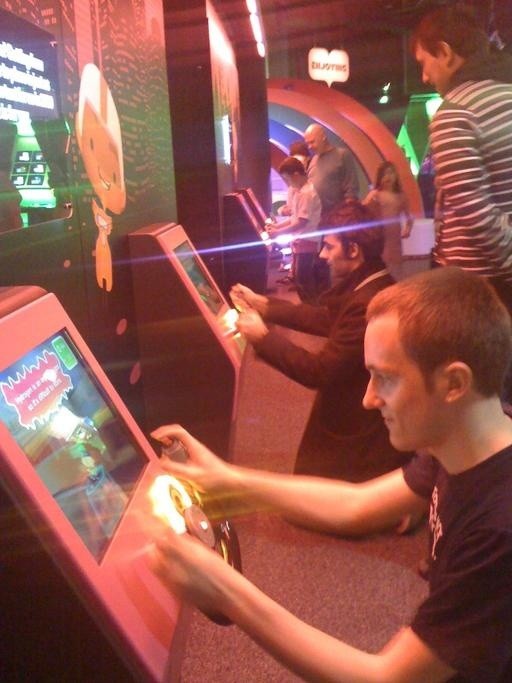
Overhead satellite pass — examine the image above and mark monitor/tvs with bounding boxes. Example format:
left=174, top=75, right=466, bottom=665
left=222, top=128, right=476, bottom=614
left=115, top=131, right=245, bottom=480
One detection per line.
left=0, top=328, right=149, bottom=564
left=6, top=134, right=60, bottom=213
left=173, top=240, right=224, bottom=317
left=242, top=191, right=267, bottom=234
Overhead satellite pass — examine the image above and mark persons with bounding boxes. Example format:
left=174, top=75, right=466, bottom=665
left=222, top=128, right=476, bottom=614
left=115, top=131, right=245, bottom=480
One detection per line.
left=228, top=198, right=428, bottom=537
left=302, top=121, right=360, bottom=218
left=142, top=264, right=512, bottom=680
left=360, top=160, right=415, bottom=280
left=265, top=157, right=323, bottom=304
left=408, top=4, right=510, bottom=407
left=416, top=151, right=437, bottom=219
left=277, top=139, right=311, bottom=215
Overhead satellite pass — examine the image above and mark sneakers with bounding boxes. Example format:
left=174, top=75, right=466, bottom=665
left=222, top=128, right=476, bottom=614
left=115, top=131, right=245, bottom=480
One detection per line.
left=397, top=502, right=429, bottom=578
